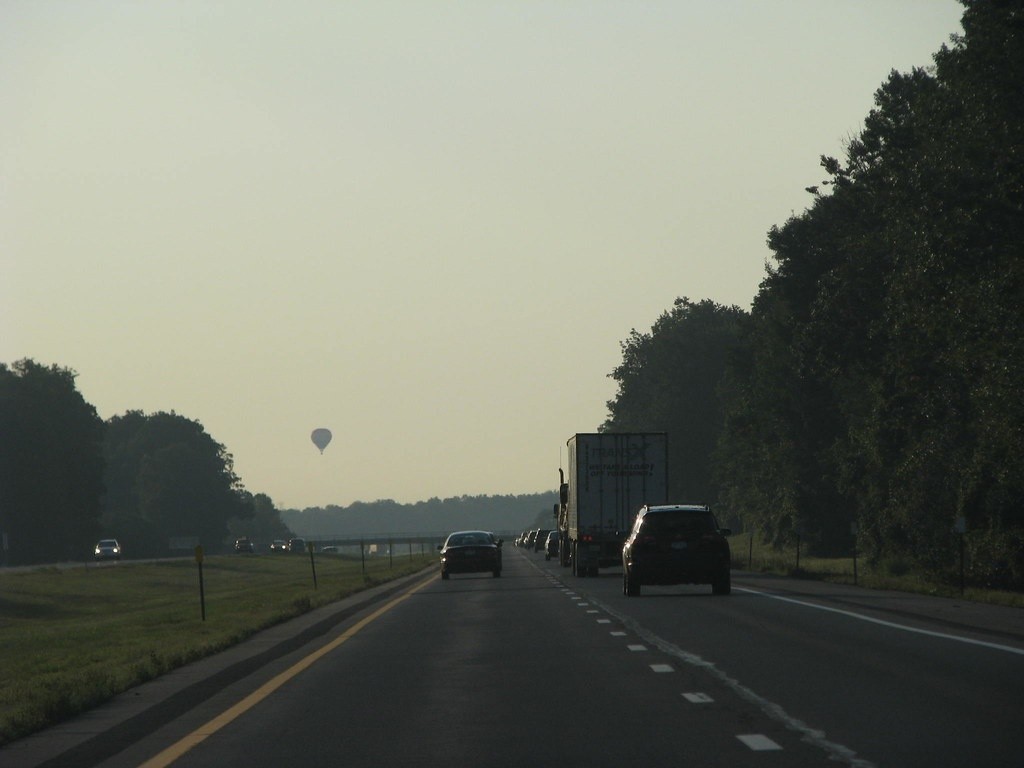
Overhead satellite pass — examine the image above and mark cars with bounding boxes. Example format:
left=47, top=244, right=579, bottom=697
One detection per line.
left=271, top=540, right=289, bottom=552
left=234, top=539, right=255, bottom=554
left=544, top=531, right=560, bottom=561
left=323, top=546, right=337, bottom=552
left=437, top=531, right=504, bottom=580
left=514, top=530, right=537, bottom=549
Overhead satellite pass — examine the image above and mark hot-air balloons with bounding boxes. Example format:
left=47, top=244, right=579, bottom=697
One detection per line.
left=311, top=428, right=332, bottom=455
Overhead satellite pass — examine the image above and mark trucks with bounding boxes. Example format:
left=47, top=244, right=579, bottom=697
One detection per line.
left=369, top=544, right=385, bottom=554
left=554, top=432, right=673, bottom=577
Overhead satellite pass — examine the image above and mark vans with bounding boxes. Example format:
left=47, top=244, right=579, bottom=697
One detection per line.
left=289, top=538, right=305, bottom=552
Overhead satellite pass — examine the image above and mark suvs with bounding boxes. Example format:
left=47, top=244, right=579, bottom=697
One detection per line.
left=95, top=539, right=121, bottom=562
left=622, top=504, right=732, bottom=597
left=534, top=528, right=557, bottom=553
